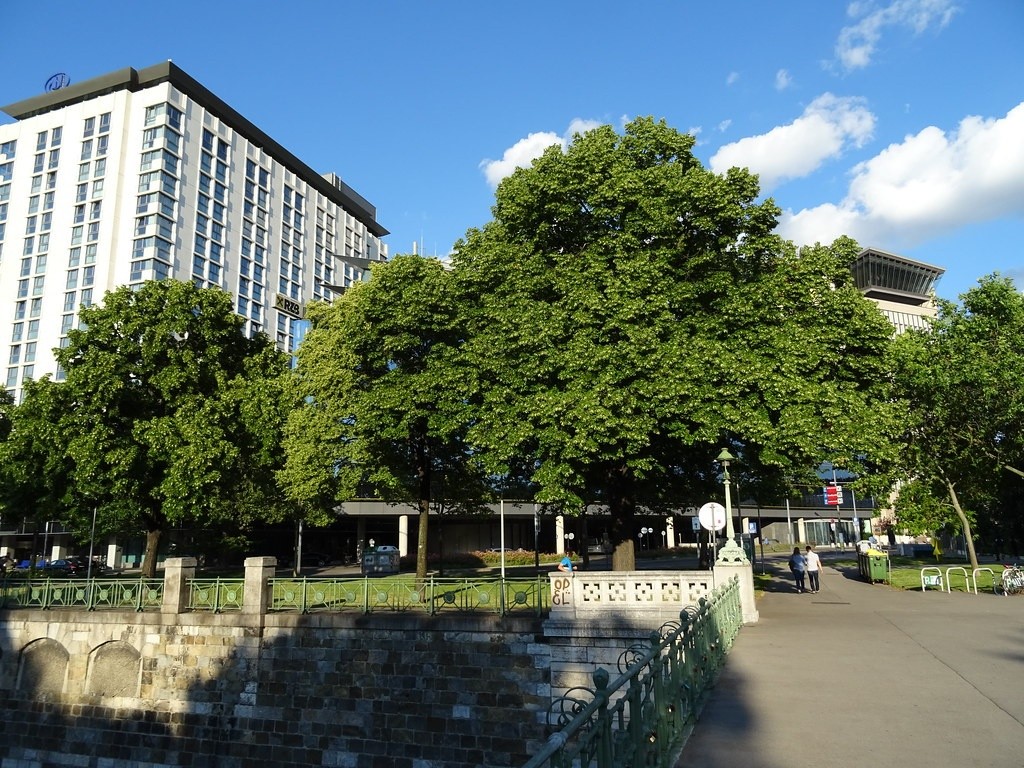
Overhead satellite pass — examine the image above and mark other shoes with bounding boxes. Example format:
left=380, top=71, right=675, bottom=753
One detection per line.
left=798, top=589, right=801, bottom=594
left=816, top=590, right=820, bottom=592
left=810, top=590, right=815, bottom=594
left=801, top=588, right=804, bottom=592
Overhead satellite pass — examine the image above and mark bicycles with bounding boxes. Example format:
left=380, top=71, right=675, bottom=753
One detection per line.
left=994, top=565, right=1024, bottom=596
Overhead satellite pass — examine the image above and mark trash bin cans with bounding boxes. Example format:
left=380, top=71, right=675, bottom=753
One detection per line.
left=362, top=545, right=400, bottom=578
left=858, top=550, right=886, bottom=584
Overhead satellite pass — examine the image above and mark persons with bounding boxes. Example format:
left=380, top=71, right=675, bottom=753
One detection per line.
left=869, top=534, right=877, bottom=544
left=557, top=548, right=577, bottom=572
left=0, top=552, right=15, bottom=587
left=789, top=547, right=807, bottom=594
left=803, top=546, right=823, bottom=593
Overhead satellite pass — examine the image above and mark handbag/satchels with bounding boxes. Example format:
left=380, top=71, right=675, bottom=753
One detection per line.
left=789, top=560, right=795, bottom=568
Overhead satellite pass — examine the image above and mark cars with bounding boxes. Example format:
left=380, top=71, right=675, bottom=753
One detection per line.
left=485, top=547, right=512, bottom=552
left=45, top=557, right=100, bottom=577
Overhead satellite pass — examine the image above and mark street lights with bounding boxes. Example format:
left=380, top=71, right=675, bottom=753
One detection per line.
left=716, top=446, right=751, bottom=567
left=85, top=490, right=98, bottom=602
